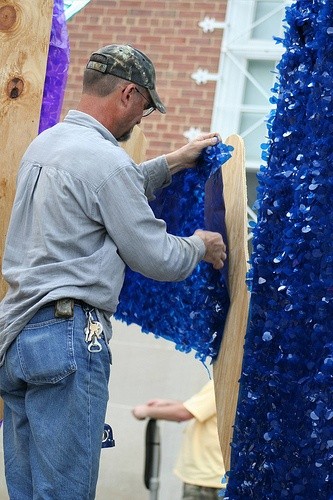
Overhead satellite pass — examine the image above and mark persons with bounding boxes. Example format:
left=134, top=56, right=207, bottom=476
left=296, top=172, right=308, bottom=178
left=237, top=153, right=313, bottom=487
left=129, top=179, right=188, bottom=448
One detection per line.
left=131, top=378, right=227, bottom=500
left=0, top=44, right=227, bottom=500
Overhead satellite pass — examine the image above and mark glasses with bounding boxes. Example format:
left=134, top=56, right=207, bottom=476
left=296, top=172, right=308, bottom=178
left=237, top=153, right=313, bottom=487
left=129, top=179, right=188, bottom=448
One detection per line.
left=122, top=87, right=156, bottom=117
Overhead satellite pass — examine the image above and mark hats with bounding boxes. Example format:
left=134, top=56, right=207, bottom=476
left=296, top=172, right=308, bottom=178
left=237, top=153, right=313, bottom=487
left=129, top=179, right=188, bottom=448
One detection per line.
left=84, top=43, right=167, bottom=114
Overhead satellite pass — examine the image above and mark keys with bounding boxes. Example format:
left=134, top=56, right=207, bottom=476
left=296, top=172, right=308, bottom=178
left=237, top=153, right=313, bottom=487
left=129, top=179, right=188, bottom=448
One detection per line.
left=84, top=310, right=102, bottom=342
